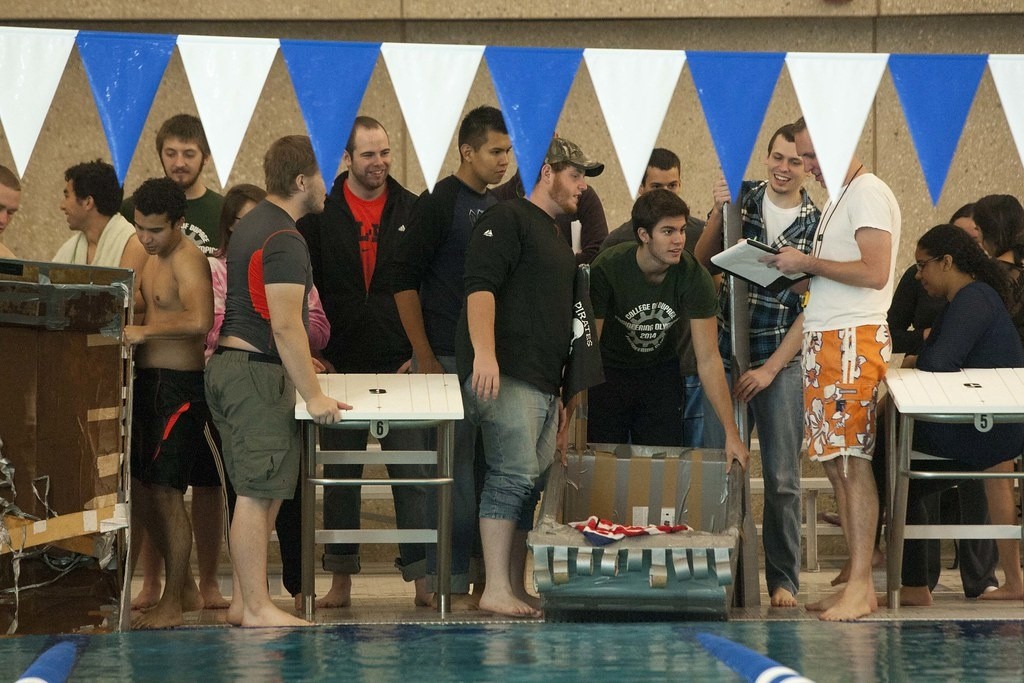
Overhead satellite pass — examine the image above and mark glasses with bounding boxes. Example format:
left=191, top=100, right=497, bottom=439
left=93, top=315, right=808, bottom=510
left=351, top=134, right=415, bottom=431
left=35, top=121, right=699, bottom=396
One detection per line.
left=916, top=254, right=945, bottom=270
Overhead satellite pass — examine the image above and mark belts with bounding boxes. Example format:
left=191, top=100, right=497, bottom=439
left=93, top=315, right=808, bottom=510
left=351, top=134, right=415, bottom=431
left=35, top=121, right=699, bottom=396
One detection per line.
left=214, top=345, right=282, bottom=365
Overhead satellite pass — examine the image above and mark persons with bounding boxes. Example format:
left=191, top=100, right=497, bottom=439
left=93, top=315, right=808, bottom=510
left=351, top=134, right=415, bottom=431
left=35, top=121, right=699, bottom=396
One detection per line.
left=47, top=104, right=901, bottom=628
left=0, top=164, right=21, bottom=260
left=885, top=193, right=1024, bottom=606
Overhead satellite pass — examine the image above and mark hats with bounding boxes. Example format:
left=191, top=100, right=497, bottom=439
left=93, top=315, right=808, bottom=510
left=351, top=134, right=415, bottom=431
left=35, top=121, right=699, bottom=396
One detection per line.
left=544, top=138, right=604, bottom=177
